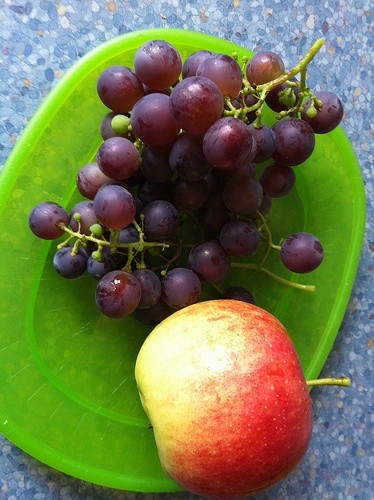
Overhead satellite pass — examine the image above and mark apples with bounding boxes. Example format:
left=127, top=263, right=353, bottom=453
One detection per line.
left=135, top=300, right=351, bottom=500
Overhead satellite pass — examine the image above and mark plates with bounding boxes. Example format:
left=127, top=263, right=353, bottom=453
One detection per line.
left=1, top=26, right=366, bottom=494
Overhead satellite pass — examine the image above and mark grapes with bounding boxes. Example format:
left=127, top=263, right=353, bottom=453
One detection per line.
left=30, top=38, right=344, bottom=325
